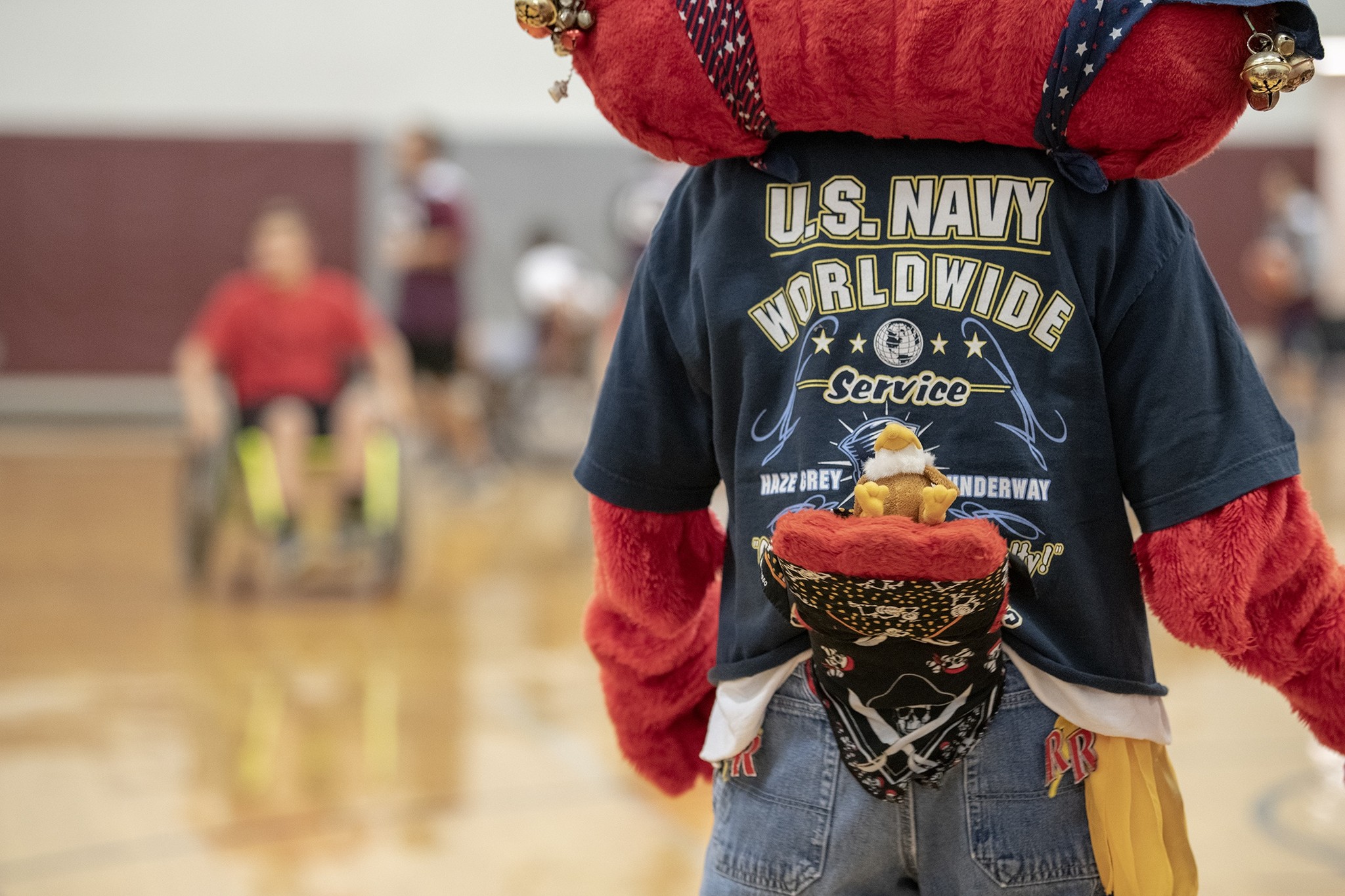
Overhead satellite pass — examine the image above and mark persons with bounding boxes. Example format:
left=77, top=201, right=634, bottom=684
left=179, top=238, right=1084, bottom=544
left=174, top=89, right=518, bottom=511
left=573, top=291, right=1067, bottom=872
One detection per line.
left=176, top=122, right=1344, bottom=545
left=515, top=0, right=1344, bottom=896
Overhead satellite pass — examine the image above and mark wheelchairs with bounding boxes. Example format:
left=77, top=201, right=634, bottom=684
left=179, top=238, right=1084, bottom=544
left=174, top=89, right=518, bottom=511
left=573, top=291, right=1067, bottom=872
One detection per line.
left=175, top=368, right=411, bottom=608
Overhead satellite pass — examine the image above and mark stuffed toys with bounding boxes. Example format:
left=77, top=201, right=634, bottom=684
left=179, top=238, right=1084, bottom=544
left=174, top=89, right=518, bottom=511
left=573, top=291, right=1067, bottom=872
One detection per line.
left=853, top=421, right=961, bottom=526
